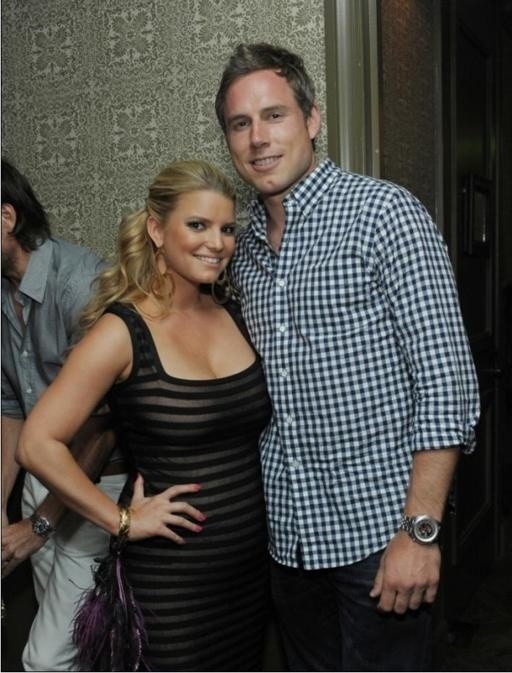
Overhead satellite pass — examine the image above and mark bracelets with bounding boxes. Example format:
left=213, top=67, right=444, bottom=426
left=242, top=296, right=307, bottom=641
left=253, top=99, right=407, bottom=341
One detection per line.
left=112, top=502, right=131, bottom=553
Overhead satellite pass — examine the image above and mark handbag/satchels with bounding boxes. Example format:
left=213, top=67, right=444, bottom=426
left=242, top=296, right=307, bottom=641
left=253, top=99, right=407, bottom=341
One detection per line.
left=67, top=555, right=146, bottom=671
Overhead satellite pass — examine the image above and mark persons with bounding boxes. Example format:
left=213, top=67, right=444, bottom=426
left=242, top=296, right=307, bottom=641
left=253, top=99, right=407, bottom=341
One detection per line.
left=15, top=158, right=278, bottom=672
left=204, top=38, right=486, bottom=670
left=0, top=156, right=131, bottom=672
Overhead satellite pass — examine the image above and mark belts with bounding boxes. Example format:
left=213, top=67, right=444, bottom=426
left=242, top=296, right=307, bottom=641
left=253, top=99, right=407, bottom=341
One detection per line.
left=98, top=461, right=130, bottom=477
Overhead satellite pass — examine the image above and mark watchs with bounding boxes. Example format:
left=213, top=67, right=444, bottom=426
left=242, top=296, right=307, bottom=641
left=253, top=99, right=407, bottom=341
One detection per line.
left=397, top=512, right=443, bottom=546
left=30, top=513, right=57, bottom=538
